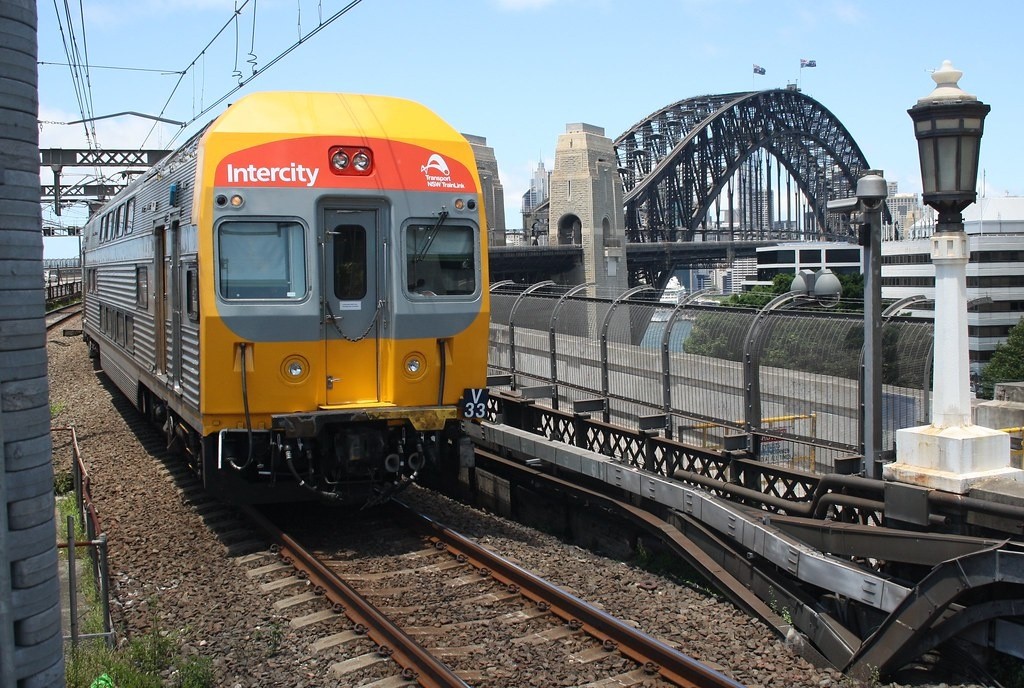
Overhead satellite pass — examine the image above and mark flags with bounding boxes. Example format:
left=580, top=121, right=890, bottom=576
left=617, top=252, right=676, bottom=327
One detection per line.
left=753, top=64, right=766, bottom=75
left=800, top=59, right=816, bottom=68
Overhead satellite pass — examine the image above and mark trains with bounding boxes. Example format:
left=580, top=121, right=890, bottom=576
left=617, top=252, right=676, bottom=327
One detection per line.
left=80, top=90, right=491, bottom=514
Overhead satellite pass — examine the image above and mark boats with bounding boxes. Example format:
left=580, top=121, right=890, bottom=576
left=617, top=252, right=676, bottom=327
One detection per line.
left=681, top=298, right=719, bottom=321
left=650, top=276, right=686, bottom=321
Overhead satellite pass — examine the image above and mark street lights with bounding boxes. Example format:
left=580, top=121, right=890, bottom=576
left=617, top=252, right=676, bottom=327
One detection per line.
left=884, top=60, right=1023, bottom=496
